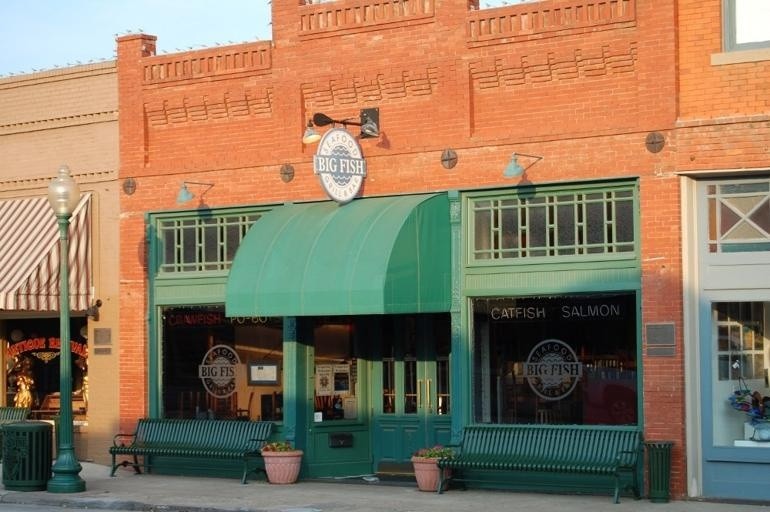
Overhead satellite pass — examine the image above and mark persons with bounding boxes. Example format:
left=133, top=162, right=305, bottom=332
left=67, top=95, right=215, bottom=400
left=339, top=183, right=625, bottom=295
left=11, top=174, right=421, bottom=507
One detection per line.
left=72, top=357, right=88, bottom=412
left=14, top=357, right=35, bottom=408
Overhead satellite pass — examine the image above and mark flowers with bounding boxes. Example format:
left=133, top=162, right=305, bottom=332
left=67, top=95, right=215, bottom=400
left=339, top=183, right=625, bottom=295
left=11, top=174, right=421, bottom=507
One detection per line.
left=413, top=445, right=456, bottom=458
left=262, top=442, right=295, bottom=452
left=728, top=376, right=770, bottom=424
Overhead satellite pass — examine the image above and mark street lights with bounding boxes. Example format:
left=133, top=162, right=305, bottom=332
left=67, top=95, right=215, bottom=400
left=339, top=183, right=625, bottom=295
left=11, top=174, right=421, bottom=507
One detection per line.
left=42, top=159, right=89, bottom=497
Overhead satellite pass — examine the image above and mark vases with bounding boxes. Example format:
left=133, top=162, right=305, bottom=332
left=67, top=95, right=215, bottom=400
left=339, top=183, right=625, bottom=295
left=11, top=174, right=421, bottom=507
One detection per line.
left=754, top=418, right=770, bottom=441
left=411, top=456, right=452, bottom=492
left=263, top=447, right=303, bottom=484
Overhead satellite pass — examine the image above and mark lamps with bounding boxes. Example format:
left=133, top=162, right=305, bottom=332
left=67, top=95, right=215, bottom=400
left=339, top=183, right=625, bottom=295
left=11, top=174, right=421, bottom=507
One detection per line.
left=502, top=152, right=542, bottom=177
left=303, top=119, right=321, bottom=143
left=176, top=180, right=215, bottom=204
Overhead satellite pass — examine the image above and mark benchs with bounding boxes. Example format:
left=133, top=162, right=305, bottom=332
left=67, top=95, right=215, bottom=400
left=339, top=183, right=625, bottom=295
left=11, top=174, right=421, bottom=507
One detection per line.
left=435, top=423, right=644, bottom=503
left=0, top=407, right=273, bottom=485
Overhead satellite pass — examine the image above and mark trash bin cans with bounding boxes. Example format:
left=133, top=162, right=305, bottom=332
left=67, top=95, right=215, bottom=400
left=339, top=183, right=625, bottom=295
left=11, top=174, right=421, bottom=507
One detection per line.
left=1, top=422, right=53, bottom=492
left=644, top=442, right=674, bottom=503
